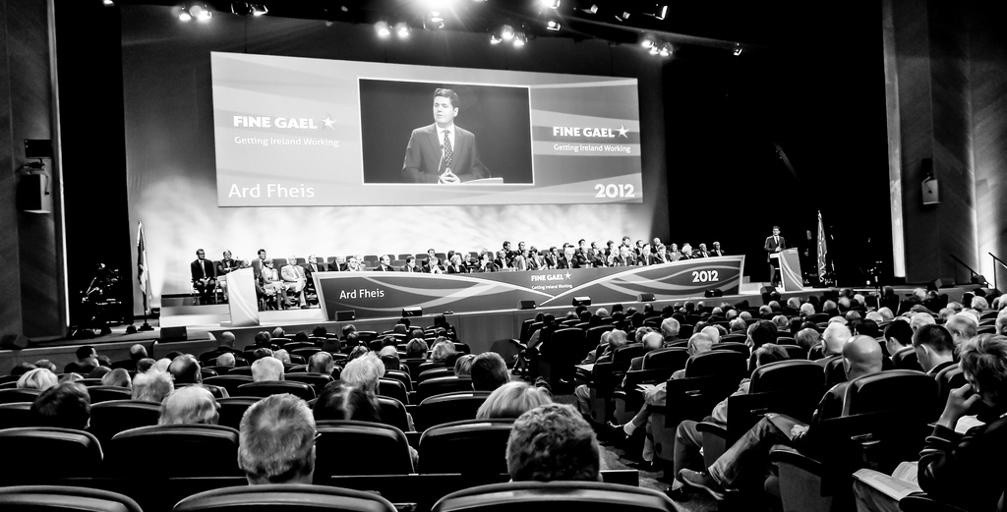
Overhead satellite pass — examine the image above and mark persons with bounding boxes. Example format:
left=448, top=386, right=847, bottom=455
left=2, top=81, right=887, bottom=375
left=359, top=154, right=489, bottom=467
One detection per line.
left=189, top=234, right=735, bottom=311
left=7, top=284, right=1007, bottom=512
left=763, top=225, right=786, bottom=287
left=399, top=86, right=483, bottom=185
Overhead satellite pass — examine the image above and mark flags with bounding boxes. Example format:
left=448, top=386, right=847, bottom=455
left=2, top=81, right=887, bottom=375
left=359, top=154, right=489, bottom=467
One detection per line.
left=816, top=211, right=834, bottom=284
left=135, top=223, right=153, bottom=311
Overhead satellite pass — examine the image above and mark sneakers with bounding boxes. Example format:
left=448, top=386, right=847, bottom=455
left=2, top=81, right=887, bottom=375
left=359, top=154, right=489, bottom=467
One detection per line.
left=679, top=467, right=727, bottom=502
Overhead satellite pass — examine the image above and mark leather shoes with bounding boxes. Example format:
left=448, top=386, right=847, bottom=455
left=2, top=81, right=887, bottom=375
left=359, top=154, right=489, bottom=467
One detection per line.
left=661, top=486, right=687, bottom=502
left=278, top=289, right=309, bottom=309
left=635, top=456, right=657, bottom=471
left=605, top=419, right=633, bottom=443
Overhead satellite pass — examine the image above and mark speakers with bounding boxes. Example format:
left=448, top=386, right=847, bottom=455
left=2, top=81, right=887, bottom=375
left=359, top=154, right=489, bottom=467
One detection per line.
left=638, top=293, right=655, bottom=301
left=19, top=173, right=52, bottom=215
left=928, top=279, right=941, bottom=291
left=572, top=296, right=591, bottom=305
left=922, top=179, right=940, bottom=205
left=941, top=277, right=955, bottom=287
left=335, top=310, right=355, bottom=321
left=401, top=307, right=422, bottom=317
left=760, top=286, right=776, bottom=293
left=520, top=300, right=536, bottom=308
left=160, top=326, right=187, bottom=341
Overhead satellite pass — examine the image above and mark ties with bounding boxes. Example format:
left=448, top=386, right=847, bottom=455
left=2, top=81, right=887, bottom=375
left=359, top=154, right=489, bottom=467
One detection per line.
left=776, top=237, right=780, bottom=245
left=292, top=266, right=300, bottom=278
left=443, top=130, right=454, bottom=169
left=313, top=263, right=318, bottom=272
